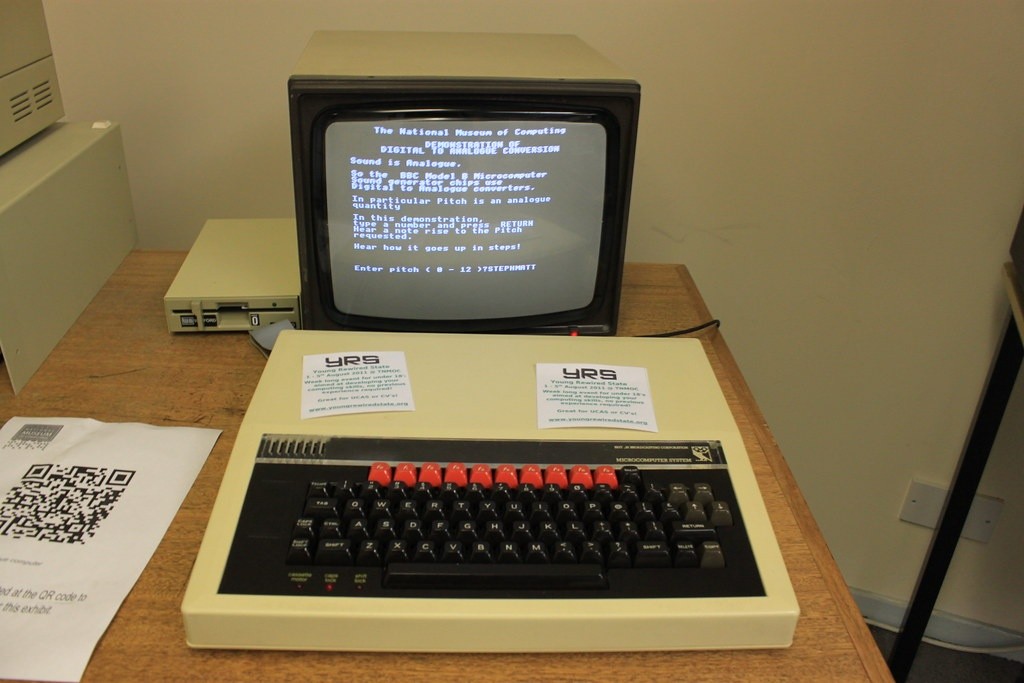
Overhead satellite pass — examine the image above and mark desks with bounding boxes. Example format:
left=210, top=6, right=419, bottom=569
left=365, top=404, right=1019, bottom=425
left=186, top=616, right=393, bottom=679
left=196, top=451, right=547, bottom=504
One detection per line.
left=0, top=249, right=898, bottom=683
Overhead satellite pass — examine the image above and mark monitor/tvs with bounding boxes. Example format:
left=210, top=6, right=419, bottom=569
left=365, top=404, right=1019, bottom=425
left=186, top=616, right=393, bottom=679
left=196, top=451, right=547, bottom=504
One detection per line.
left=283, top=26, right=641, bottom=336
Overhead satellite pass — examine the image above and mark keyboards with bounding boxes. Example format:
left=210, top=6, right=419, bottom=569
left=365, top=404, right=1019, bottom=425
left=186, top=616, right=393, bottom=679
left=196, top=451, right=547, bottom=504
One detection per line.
left=217, top=434, right=768, bottom=596
